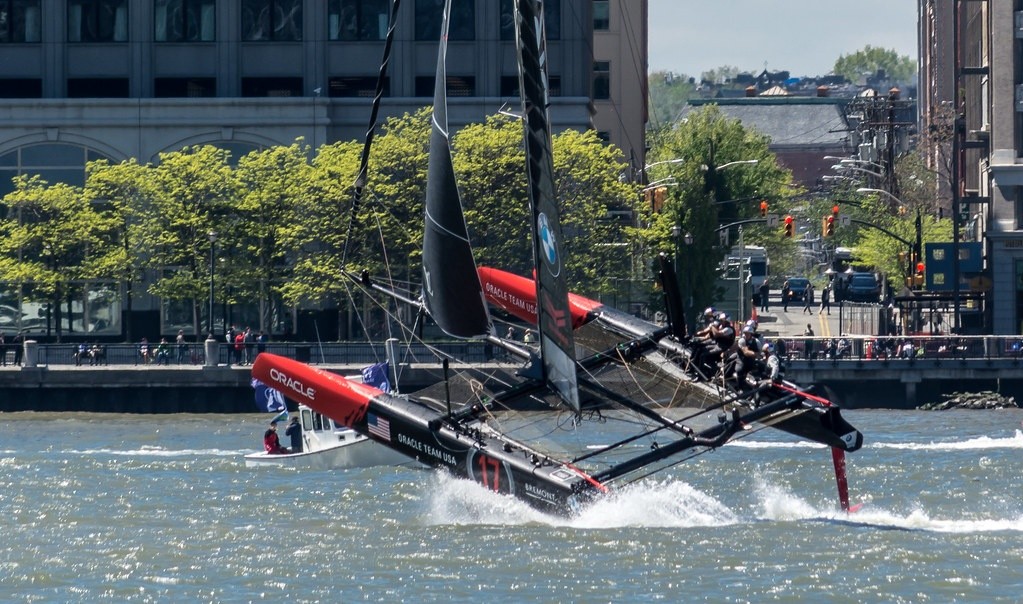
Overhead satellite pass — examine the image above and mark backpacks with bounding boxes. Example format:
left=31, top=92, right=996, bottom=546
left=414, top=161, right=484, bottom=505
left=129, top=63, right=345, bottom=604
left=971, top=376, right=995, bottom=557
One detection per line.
left=915, top=346, right=926, bottom=358
left=805, top=330, right=812, bottom=344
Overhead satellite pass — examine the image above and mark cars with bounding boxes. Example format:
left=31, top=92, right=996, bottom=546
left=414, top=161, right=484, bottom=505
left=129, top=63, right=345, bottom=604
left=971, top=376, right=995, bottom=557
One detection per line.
left=781, top=278, right=814, bottom=303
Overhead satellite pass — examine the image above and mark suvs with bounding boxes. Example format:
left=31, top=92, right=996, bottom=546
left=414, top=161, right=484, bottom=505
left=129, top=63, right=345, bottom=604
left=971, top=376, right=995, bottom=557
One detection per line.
left=846, top=273, right=878, bottom=304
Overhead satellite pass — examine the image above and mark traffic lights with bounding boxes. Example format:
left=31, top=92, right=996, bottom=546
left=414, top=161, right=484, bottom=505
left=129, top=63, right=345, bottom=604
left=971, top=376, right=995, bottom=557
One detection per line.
left=761, top=203, right=767, bottom=218
left=917, top=264, right=924, bottom=271
left=834, top=206, right=838, bottom=218
left=785, top=216, right=792, bottom=237
left=825, top=215, right=835, bottom=235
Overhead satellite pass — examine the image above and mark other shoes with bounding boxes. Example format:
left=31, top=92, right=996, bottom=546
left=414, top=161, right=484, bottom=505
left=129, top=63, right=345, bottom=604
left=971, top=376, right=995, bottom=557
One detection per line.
left=683, top=369, right=701, bottom=383
left=0, top=363, right=21, bottom=367
left=739, top=400, right=749, bottom=406
left=737, top=388, right=743, bottom=395
left=138, top=359, right=185, bottom=366
left=236, top=361, right=251, bottom=366
left=76, top=362, right=109, bottom=368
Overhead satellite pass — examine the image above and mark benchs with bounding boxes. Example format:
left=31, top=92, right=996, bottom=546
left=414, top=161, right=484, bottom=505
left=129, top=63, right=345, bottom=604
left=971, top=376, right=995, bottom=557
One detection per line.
left=786, top=341, right=827, bottom=359
left=870, top=345, right=917, bottom=359
left=924, top=340, right=972, bottom=358
left=75, top=347, right=108, bottom=366
left=138, top=348, right=175, bottom=365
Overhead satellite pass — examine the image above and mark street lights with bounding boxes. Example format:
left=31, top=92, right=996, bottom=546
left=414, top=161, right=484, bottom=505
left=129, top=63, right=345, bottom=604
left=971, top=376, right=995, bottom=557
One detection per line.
left=207, top=230, right=217, bottom=339
left=672, top=224, right=680, bottom=272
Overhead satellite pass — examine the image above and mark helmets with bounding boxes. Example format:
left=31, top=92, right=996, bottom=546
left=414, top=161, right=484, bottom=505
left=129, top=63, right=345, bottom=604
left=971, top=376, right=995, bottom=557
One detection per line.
left=742, top=319, right=756, bottom=334
left=762, top=343, right=774, bottom=352
left=703, top=307, right=731, bottom=323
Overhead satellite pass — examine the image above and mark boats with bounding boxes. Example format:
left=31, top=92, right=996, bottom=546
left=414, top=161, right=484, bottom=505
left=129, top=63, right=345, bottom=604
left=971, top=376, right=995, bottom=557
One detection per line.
left=242, top=373, right=416, bottom=470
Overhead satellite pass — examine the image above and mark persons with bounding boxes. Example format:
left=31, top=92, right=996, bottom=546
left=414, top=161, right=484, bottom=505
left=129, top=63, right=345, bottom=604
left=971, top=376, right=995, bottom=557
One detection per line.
left=157, top=338, right=171, bottom=364
left=945, top=337, right=968, bottom=358
left=872, top=332, right=914, bottom=359
left=140, top=337, right=148, bottom=363
left=687, top=307, right=780, bottom=412
left=226, top=326, right=266, bottom=366
left=0, top=333, right=6, bottom=366
left=264, top=422, right=289, bottom=454
left=12, top=332, right=23, bottom=366
left=73, top=341, right=102, bottom=365
left=825, top=335, right=851, bottom=359
left=524, top=328, right=534, bottom=345
left=176, top=329, right=186, bottom=363
left=805, top=323, right=813, bottom=358
left=286, top=416, right=302, bottom=453
left=1012, top=340, right=1023, bottom=357
left=781, top=281, right=791, bottom=312
left=505, top=327, right=516, bottom=364
left=803, top=284, right=832, bottom=315
left=759, top=280, right=769, bottom=312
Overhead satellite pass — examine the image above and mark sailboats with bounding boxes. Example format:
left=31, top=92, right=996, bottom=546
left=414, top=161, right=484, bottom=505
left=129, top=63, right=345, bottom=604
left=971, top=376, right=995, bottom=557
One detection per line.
left=254, top=0, right=861, bottom=517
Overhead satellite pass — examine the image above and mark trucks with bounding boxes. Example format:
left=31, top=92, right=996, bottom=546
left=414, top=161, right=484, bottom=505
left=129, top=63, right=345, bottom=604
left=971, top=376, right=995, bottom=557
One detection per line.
left=730, top=245, right=769, bottom=305
left=832, top=247, right=875, bottom=301
